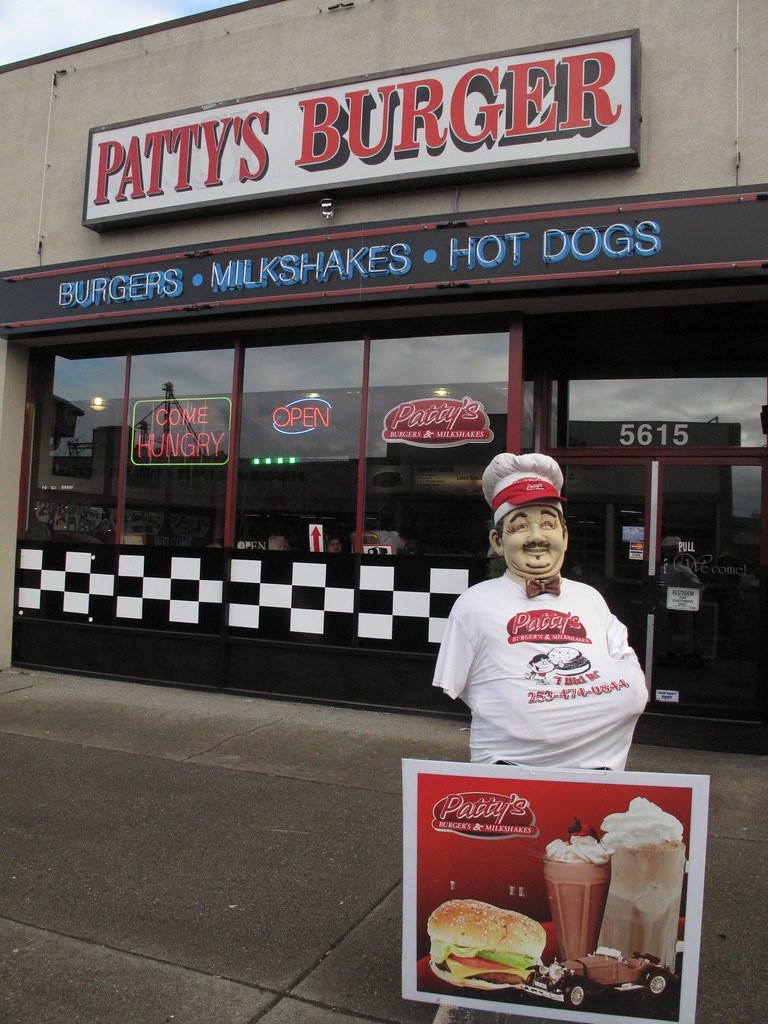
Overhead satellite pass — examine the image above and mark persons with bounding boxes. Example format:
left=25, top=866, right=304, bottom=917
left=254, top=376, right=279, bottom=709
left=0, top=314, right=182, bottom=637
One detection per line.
left=706, top=546, right=743, bottom=601
left=270, top=536, right=289, bottom=550
left=433, top=452, right=649, bottom=769
left=662, top=536, right=705, bottom=652
left=325, top=535, right=343, bottom=552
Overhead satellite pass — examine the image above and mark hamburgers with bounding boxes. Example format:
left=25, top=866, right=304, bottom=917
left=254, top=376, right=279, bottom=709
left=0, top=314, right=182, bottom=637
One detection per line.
left=428, top=900, right=547, bottom=990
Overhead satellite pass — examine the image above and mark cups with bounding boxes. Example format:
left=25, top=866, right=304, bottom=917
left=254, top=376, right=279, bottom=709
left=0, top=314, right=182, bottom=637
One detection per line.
left=541, top=861, right=600, bottom=962
left=600, top=843, right=686, bottom=977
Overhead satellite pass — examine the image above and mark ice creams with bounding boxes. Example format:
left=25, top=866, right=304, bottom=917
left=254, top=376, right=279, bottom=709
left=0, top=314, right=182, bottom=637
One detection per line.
left=541, top=797, right=686, bottom=973
